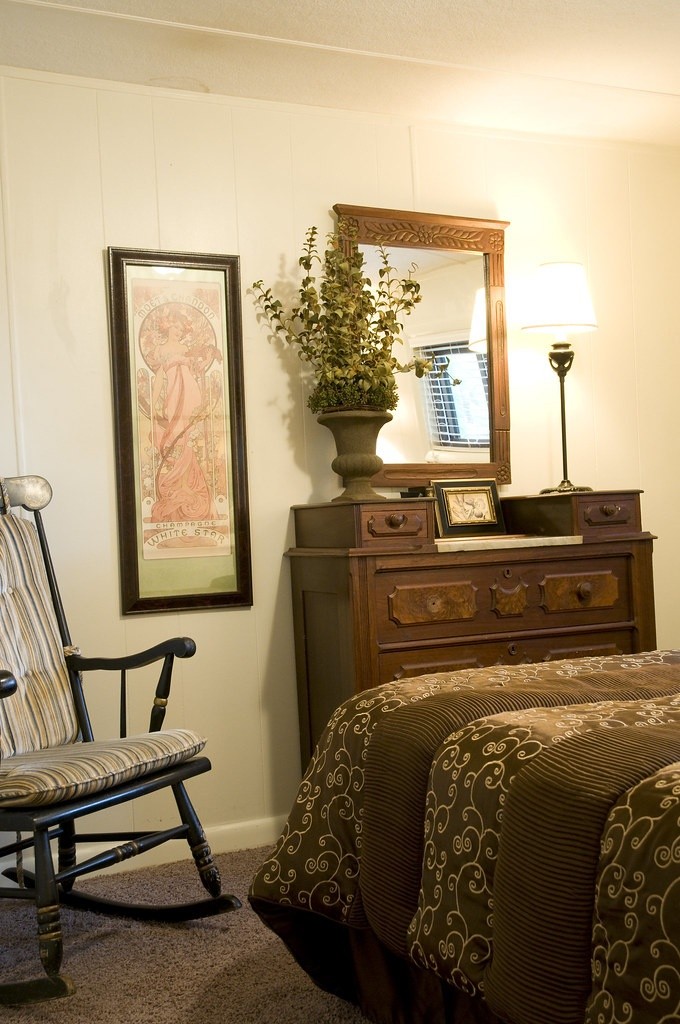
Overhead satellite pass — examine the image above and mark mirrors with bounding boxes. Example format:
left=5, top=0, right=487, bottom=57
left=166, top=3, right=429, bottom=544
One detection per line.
left=332, top=203, right=512, bottom=487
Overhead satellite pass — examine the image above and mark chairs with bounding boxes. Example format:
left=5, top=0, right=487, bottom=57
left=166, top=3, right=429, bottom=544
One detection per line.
left=1, top=474, right=243, bottom=1007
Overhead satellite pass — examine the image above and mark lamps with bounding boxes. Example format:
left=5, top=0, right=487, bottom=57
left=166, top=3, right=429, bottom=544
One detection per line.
left=520, top=260, right=598, bottom=496
left=466, top=285, right=488, bottom=353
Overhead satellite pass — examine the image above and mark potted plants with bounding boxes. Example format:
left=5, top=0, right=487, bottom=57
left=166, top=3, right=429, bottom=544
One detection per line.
left=252, top=214, right=464, bottom=503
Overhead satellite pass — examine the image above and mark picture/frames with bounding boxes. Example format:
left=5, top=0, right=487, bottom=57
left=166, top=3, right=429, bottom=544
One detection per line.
left=108, top=246, right=254, bottom=615
left=429, top=479, right=507, bottom=537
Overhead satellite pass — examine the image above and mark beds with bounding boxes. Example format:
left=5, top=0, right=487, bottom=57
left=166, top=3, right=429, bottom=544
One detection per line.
left=248, top=649, right=680, bottom=1023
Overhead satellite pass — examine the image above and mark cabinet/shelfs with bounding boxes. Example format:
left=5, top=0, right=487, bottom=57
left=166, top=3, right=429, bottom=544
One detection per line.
left=283, top=489, right=657, bottom=777
